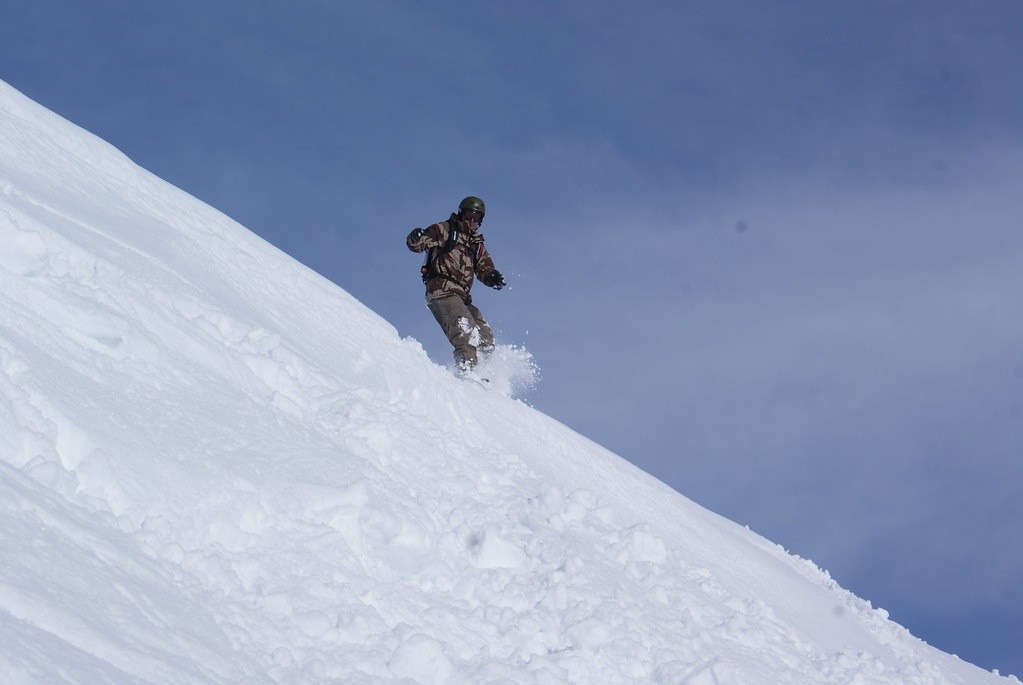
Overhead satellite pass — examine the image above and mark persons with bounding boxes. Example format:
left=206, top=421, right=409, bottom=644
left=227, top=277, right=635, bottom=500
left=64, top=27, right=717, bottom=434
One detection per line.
left=406, top=196, right=506, bottom=370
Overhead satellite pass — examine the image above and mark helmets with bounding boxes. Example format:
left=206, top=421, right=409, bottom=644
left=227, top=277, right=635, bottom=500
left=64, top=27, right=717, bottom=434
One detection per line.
left=459, top=196, right=485, bottom=214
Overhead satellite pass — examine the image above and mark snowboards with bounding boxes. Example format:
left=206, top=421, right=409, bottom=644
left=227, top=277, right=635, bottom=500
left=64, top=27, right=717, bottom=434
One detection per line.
left=458, top=371, right=487, bottom=392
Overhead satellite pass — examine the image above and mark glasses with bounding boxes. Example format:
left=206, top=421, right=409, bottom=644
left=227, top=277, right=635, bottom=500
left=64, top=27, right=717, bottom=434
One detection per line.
left=462, top=209, right=483, bottom=223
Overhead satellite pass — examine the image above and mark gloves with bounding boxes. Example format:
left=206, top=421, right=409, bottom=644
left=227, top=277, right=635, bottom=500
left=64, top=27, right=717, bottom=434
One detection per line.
left=411, top=228, right=425, bottom=244
left=490, top=271, right=506, bottom=290
